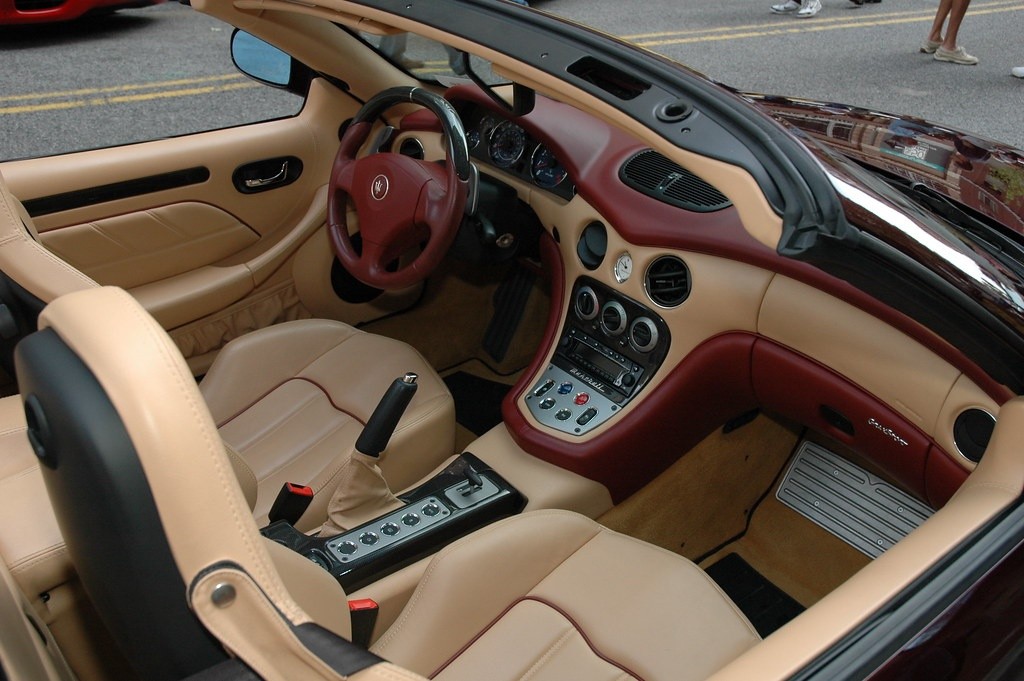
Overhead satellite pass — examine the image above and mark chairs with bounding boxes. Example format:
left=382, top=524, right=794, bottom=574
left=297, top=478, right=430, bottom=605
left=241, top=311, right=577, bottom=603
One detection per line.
left=13, top=284, right=763, bottom=681
left=0, top=170, right=456, bottom=533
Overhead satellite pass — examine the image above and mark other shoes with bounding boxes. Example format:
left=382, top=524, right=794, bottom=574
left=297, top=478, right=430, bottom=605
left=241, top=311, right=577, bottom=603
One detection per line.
left=1012, top=66, right=1024, bottom=77
left=848, top=0, right=863, bottom=8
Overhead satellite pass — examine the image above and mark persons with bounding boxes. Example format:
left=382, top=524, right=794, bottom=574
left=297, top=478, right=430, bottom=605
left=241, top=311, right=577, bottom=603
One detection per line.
left=887, top=120, right=991, bottom=171
left=919, top=0, right=979, bottom=64
left=770, top=0, right=822, bottom=18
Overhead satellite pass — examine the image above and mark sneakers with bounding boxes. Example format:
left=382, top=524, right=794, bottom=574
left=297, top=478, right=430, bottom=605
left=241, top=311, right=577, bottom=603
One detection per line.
left=770, top=0, right=801, bottom=14
left=933, top=46, right=978, bottom=64
left=797, top=0, right=822, bottom=18
left=920, top=36, right=945, bottom=53
